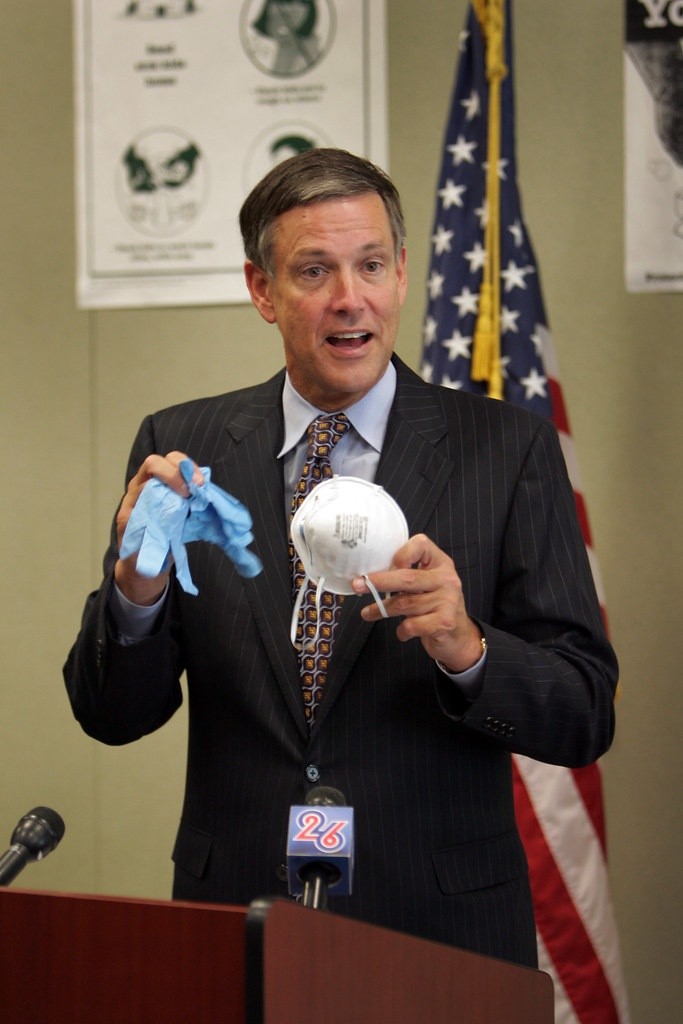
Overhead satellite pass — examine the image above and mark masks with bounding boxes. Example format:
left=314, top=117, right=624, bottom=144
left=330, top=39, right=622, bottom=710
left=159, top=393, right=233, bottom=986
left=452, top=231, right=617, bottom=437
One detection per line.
left=289, top=477, right=409, bottom=650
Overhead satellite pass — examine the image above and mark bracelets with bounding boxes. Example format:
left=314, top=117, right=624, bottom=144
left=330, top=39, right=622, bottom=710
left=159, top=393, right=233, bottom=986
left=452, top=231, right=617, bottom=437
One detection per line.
left=478, top=635, right=487, bottom=654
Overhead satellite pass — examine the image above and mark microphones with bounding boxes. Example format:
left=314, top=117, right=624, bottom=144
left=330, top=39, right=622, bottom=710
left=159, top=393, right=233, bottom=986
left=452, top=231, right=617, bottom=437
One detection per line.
left=286, top=787, right=357, bottom=913
left=0, top=806, right=65, bottom=888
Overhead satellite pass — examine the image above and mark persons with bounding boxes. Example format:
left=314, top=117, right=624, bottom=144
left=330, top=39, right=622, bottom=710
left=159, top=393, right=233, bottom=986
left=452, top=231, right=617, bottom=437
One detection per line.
left=62, top=149, right=622, bottom=972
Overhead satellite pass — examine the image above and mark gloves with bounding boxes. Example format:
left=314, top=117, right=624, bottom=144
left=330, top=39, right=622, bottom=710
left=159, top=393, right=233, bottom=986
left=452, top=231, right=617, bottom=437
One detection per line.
left=118, top=458, right=265, bottom=596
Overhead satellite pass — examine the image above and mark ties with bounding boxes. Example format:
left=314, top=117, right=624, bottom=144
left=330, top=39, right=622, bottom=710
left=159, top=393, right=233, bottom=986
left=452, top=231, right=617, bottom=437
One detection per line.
left=289, top=415, right=354, bottom=732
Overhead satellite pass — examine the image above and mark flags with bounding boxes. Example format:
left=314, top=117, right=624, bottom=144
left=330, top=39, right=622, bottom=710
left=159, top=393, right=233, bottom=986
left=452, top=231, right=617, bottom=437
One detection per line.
left=419, top=0, right=633, bottom=1024
